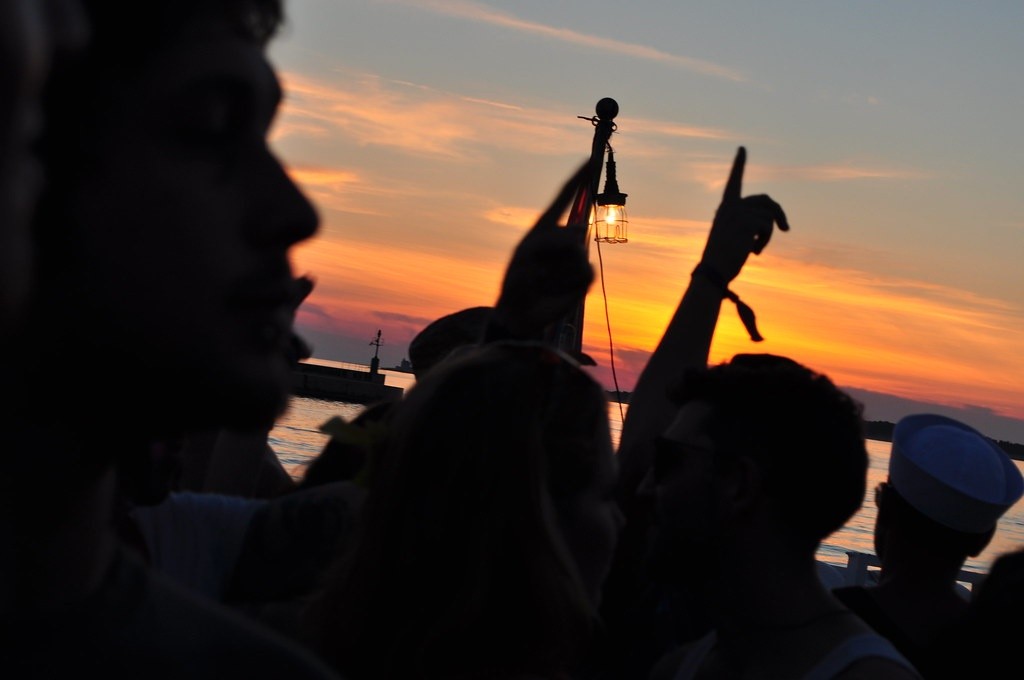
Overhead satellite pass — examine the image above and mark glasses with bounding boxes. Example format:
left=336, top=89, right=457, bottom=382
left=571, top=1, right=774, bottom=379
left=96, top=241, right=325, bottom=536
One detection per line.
left=651, top=431, right=773, bottom=496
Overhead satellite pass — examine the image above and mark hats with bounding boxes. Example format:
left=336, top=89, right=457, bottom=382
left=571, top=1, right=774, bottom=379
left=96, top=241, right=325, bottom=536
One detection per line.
left=891, top=414, right=1023, bottom=533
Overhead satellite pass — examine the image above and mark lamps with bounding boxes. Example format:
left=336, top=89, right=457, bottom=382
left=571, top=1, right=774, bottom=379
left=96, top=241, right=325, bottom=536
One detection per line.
left=592, top=146, right=629, bottom=243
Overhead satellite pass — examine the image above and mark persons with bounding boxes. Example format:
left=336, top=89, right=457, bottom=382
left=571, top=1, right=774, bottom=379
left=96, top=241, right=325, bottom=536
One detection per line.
left=0, top=0, right=1024, bottom=680
